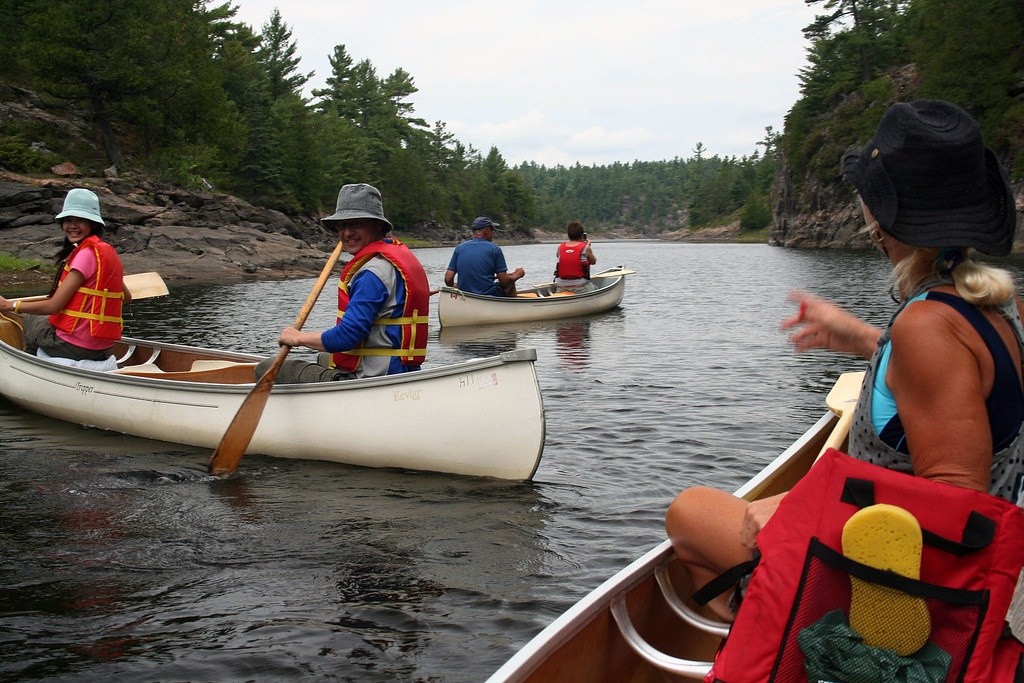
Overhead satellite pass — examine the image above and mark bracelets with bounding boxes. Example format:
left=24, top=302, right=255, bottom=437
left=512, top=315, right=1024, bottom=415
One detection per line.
left=12, top=300, right=22, bottom=314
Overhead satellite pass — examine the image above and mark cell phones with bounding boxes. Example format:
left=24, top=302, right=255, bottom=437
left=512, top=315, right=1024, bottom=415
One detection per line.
left=583, top=233, right=587, bottom=242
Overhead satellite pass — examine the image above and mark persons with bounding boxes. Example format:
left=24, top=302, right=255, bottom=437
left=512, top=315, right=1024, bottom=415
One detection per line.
left=253, top=183, right=432, bottom=386
left=443, top=216, right=526, bottom=297
left=554, top=221, right=596, bottom=280
left=665, top=97, right=1023, bottom=623
left=0, top=188, right=132, bottom=362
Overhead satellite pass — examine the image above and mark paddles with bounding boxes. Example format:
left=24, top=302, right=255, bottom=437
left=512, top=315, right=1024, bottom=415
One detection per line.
left=812, top=371, right=875, bottom=479
left=207, top=241, right=345, bottom=475
left=533, top=268, right=634, bottom=282
left=0, top=271, right=170, bottom=310
left=429, top=271, right=523, bottom=297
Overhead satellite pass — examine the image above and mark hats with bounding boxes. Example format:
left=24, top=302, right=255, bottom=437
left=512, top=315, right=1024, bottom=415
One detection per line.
left=55, top=188, right=104, bottom=225
left=321, top=183, right=392, bottom=232
left=841, top=99, right=1018, bottom=255
left=471, top=216, right=500, bottom=230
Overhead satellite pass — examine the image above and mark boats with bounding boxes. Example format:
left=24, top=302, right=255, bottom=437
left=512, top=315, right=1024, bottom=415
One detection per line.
left=0, top=294, right=546, bottom=482
left=438, top=263, right=626, bottom=327
